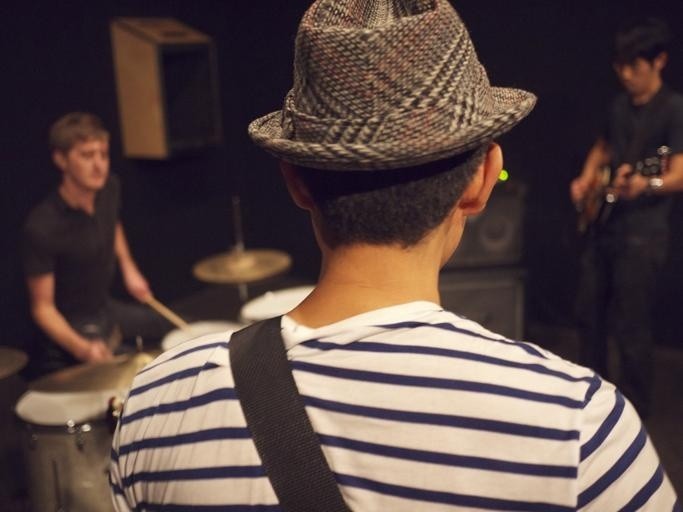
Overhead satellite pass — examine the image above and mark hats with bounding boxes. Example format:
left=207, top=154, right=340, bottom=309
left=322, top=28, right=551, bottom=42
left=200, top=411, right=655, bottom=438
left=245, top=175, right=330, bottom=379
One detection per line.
left=246, top=2, right=539, bottom=170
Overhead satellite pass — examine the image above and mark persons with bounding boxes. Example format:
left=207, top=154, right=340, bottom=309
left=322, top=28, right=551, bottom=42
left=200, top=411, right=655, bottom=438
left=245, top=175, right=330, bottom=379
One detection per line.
left=107, top=0, right=678, bottom=511
left=21, top=110, right=174, bottom=377
left=569, top=25, right=683, bottom=419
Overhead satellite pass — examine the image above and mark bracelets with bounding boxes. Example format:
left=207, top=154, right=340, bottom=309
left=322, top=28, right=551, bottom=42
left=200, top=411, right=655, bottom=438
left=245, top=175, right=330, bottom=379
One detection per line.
left=649, top=176, right=664, bottom=196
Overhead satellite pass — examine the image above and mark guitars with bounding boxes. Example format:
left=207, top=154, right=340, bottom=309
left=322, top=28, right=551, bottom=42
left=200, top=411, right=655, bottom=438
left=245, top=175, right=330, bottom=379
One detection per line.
left=576, top=146, right=671, bottom=237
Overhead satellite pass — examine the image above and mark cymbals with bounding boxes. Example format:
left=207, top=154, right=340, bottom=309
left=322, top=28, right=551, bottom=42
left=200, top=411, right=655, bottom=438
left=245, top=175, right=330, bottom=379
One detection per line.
left=236, top=286, right=315, bottom=324
left=2, top=344, right=29, bottom=377
left=191, top=247, right=289, bottom=285
left=163, top=318, right=236, bottom=350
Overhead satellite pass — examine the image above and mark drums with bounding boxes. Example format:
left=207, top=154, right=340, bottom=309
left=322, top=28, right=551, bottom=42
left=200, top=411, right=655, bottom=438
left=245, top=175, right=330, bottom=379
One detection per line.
left=20, top=353, right=149, bottom=512
